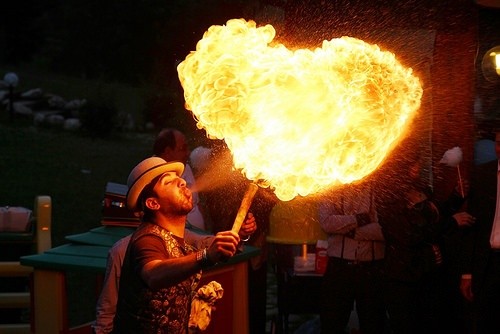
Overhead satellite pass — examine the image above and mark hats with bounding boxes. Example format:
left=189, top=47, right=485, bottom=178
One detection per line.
left=124, top=157, right=185, bottom=213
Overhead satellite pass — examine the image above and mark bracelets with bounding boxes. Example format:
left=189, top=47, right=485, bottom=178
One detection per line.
left=240, top=234, right=250, bottom=242
left=195, top=247, right=208, bottom=264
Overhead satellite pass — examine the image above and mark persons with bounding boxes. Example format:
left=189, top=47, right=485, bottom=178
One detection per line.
left=266, top=123, right=500, bottom=334
left=91, top=156, right=258, bottom=334
left=149, top=127, right=206, bottom=230
left=191, top=138, right=276, bottom=334
left=91, top=212, right=258, bottom=334
